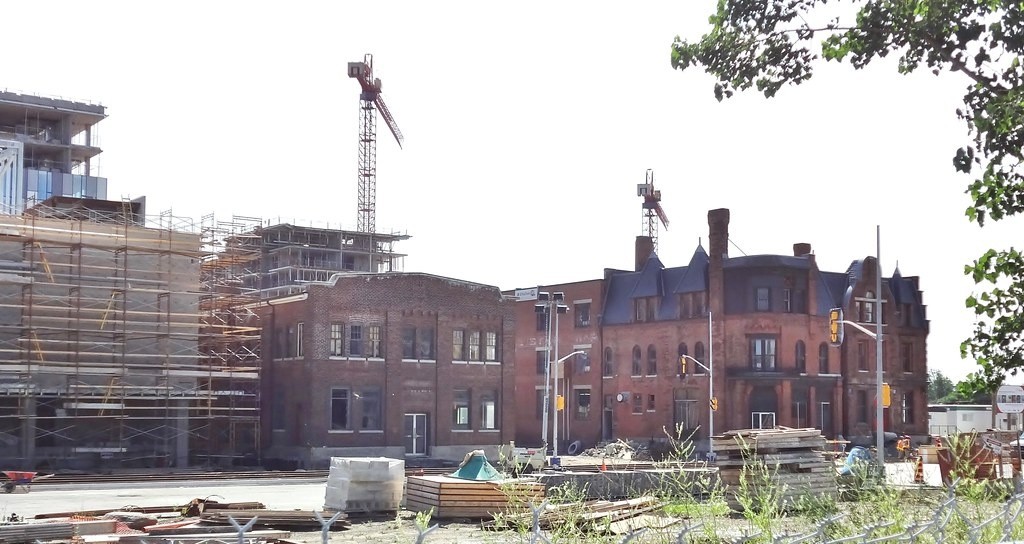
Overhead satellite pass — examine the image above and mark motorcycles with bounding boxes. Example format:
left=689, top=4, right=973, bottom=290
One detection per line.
left=897, top=431, right=911, bottom=458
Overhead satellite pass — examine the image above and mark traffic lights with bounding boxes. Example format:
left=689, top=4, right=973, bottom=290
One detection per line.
left=709, top=396, right=718, bottom=412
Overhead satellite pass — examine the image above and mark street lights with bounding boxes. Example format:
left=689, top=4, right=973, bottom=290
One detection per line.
left=534, top=292, right=587, bottom=471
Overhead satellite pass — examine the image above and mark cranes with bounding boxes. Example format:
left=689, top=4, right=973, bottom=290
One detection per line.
left=637, top=169, right=669, bottom=255
left=347, top=54, right=403, bottom=249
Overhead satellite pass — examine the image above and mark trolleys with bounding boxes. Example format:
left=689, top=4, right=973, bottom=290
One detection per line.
left=1, top=469, right=55, bottom=494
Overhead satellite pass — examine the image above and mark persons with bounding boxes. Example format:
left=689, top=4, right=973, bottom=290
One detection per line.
left=897, top=431, right=912, bottom=461
